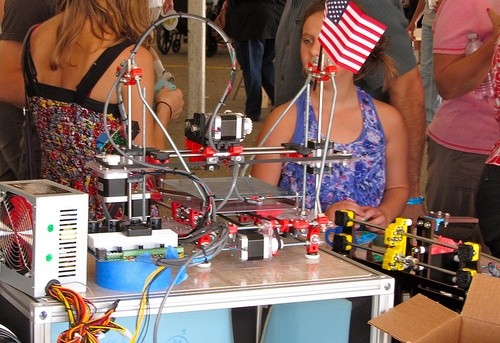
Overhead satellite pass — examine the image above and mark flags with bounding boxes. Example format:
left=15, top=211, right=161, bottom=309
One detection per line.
left=318, top=0, right=386, bottom=74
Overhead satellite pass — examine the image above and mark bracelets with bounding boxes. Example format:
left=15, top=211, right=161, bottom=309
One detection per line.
left=155, top=101, right=173, bottom=119
left=407, top=198, right=422, bottom=205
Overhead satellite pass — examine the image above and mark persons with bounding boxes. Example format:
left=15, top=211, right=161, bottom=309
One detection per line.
left=21, top=0, right=185, bottom=224
left=251, top=7, right=411, bottom=258
left=275, top=0, right=426, bottom=248
left=408, top=0, right=442, bottom=142
left=0, top=0, right=69, bottom=182
left=224, top=0, right=287, bottom=121
left=427, top=0, right=500, bottom=259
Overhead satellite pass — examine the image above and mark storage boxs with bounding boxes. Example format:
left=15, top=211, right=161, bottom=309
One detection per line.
left=367, top=273, right=500, bottom=343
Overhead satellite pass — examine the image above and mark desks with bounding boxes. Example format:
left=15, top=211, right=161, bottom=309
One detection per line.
left=0, top=187, right=396, bottom=343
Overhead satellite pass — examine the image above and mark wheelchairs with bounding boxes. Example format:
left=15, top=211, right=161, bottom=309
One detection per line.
left=157, top=0, right=217, bottom=59
